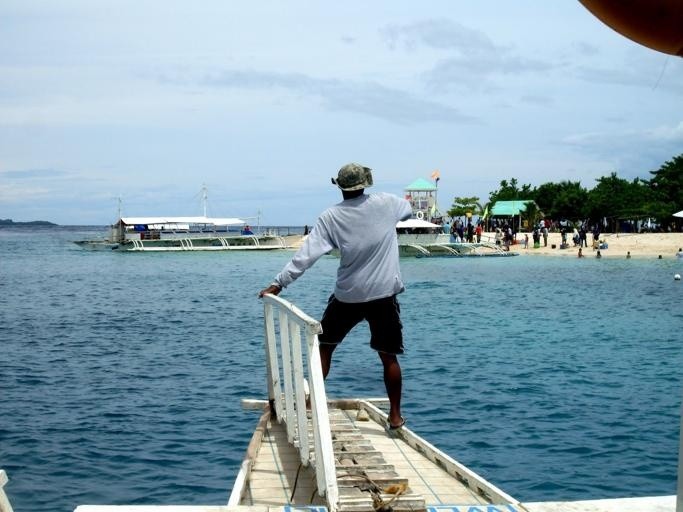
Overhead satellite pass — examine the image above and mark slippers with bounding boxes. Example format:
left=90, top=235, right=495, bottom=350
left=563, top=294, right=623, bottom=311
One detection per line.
left=388, top=414, right=405, bottom=429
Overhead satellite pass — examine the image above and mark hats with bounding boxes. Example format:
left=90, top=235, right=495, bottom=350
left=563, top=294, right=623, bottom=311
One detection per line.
left=330, top=161, right=373, bottom=194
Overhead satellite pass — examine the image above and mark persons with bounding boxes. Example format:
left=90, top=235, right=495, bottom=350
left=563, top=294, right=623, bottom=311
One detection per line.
left=626, top=251, right=631, bottom=259
left=245, top=225, right=249, bottom=231
left=674, top=274, right=680, bottom=280
left=442, top=216, right=683, bottom=251
left=658, top=255, right=662, bottom=258
left=260, top=164, right=412, bottom=428
left=597, top=251, right=601, bottom=258
left=578, top=249, right=583, bottom=257
left=676, top=248, right=683, bottom=258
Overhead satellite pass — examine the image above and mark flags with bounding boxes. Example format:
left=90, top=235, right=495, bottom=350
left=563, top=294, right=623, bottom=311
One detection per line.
left=432, top=169, right=440, bottom=181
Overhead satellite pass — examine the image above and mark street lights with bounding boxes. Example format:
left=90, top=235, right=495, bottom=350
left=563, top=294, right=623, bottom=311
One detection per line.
left=434, top=177, right=441, bottom=218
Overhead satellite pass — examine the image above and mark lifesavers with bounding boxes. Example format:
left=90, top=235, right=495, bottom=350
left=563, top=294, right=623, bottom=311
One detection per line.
left=417, top=211, right=424, bottom=220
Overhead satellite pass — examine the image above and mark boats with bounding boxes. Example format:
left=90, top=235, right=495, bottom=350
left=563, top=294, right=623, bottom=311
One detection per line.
left=67, top=183, right=274, bottom=250
